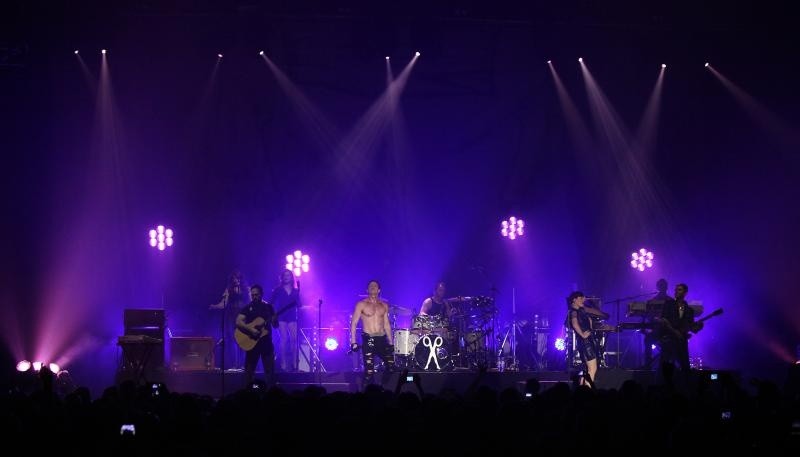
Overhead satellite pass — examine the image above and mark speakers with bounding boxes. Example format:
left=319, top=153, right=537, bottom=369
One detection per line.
left=170, top=336, right=217, bottom=372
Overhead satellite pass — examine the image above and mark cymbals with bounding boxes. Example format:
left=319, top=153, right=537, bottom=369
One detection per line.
left=583, top=307, right=610, bottom=320
left=593, top=323, right=619, bottom=333
left=447, top=296, right=479, bottom=301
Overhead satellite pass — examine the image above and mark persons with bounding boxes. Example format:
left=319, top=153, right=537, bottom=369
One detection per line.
left=419, top=282, right=451, bottom=318
left=0, top=364, right=800, bottom=457
left=640, top=279, right=705, bottom=370
left=350, top=279, right=393, bottom=389
left=564, top=291, right=597, bottom=388
left=209, top=269, right=302, bottom=386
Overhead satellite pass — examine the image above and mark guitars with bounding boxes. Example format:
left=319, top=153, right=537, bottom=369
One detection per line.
left=669, top=308, right=723, bottom=341
left=234, top=301, right=299, bottom=350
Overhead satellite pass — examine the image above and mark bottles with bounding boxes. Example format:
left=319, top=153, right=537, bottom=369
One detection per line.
left=152, top=384, right=159, bottom=398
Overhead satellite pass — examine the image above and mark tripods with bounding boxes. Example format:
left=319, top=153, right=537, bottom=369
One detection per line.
left=498, top=288, right=540, bottom=372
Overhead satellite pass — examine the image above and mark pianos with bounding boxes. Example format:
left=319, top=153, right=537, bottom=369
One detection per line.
left=621, top=302, right=703, bottom=370
left=117, top=334, right=162, bottom=389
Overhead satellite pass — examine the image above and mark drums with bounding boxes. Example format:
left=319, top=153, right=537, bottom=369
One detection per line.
left=410, top=315, right=450, bottom=333
left=393, top=329, right=421, bottom=355
left=414, top=334, right=451, bottom=372
left=463, top=331, right=486, bottom=352
